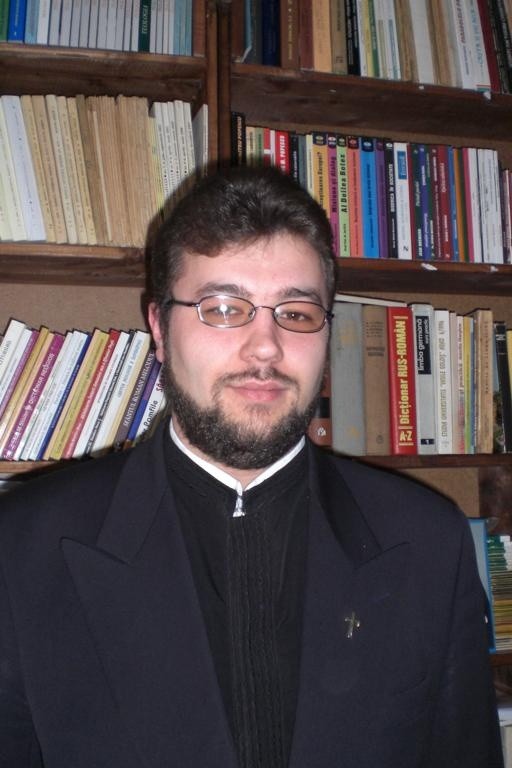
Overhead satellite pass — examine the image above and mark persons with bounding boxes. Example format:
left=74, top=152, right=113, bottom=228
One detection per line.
left=1, top=157, right=503, bottom=768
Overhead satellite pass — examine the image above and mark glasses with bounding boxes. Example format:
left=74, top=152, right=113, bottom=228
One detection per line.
left=161, top=296, right=334, bottom=333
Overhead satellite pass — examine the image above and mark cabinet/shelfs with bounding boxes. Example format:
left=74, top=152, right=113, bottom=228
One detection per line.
left=0, top=0, right=512, bottom=711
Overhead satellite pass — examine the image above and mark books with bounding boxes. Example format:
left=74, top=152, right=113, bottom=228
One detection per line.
left=0, top=293, right=511, bottom=462
left=464, top=513, right=511, bottom=652
left=0, top=94, right=512, bottom=265
left=496, top=695, right=511, bottom=768
left=1, top=1, right=511, bottom=91
